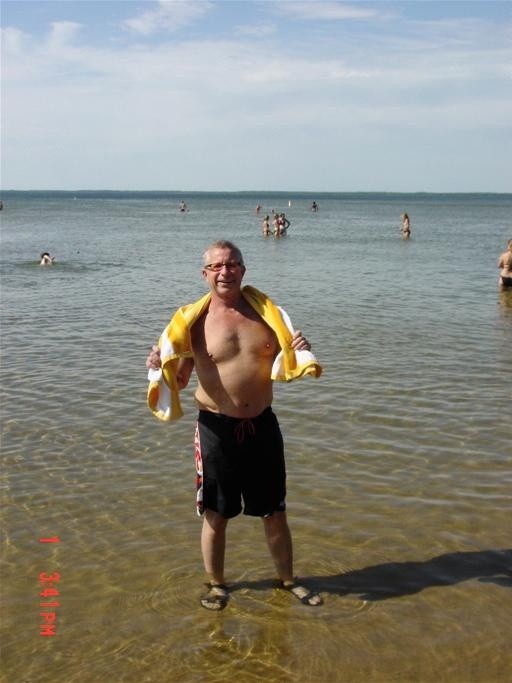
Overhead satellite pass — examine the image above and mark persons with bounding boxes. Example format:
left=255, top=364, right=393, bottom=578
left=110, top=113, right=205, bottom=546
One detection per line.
left=177, top=198, right=319, bottom=241
left=39, top=251, right=56, bottom=266
left=147, top=241, right=324, bottom=611
left=399, top=213, right=411, bottom=241
left=496, top=238, right=512, bottom=293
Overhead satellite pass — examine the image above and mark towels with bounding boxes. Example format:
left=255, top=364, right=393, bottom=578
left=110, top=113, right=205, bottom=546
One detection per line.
left=146, top=285, right=322, bottom=424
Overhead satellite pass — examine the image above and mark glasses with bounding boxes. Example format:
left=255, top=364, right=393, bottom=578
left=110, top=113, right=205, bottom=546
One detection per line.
left=204, top=262, right=240, bottom=272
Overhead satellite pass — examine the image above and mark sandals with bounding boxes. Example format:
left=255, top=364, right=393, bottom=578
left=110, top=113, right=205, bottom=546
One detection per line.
left=282, top=578, right=323, bottom=605
left=200, top=583, right=227, bottom=611
